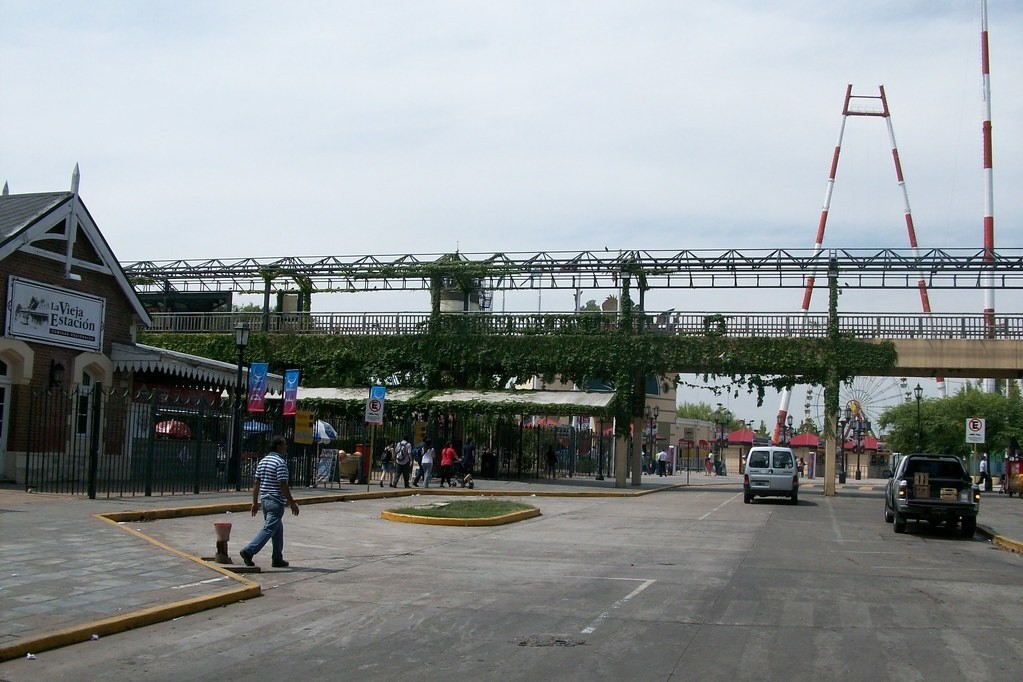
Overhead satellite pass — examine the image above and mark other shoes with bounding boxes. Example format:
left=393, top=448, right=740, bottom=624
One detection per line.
left=425, top=485, right=431, bottom=488
left=272, top=559, right=289, bottom=567
left=393, top=484, right=396, bottom=488
left=440, top=485, right=444, bottom=487
left=240, top=549, right=255, bottom=566
left=405, top=485, right=411, bottom=488
left=413, top=482, right=418, bottom=487
left=379, top=481, right=383, bottom=487
left=449, top=483, right=452, bottom=487
left=390, top=484, right=393, bottom=487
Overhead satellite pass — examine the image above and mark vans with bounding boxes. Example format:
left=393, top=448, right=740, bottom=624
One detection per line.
left=743, top=444, right=800, bottom=506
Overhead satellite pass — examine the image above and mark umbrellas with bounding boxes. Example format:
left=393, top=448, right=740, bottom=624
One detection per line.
left=314, top=418, right=338, bottom=484
left=155, top=418, right=192, bottom=437
left=244, top=421, right=272, bottom=433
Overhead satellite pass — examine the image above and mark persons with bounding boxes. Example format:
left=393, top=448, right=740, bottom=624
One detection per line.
left=461, top=437, right=476, bottom=489
left=380, top=434, right=436, bottom=488
left=641, top=449, right=668, bottom=477
left=796, top=456, right=806, bottom=477
left=976, top=456, right=987, bottom=486
left=239, top=435, right=299, bottom=567
left=704, top=450, right=716, bottom=476
left=544, top=445, right=558, bottom=479
left=440, top=441, right=460, bottom=487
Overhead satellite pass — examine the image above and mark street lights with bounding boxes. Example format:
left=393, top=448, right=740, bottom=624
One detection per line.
left=229, top=320, right=251, bottom=484
left=595, top=411, right=605, bottom=481
left=644, top=403, right=659, bottom=475
left=836, top=406, right=852, bottom=484
left=850, top=416, right=869, bottom=480
left=714, top=409, right=732, bottom=475
left=914, top=381, right=923, bottom=453
left=686, top=431, right=691, bottom=484
left=777, top=413, right=794, bottom=447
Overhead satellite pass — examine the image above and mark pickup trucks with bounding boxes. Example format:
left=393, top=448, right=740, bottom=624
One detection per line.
left=882, top=453, right=981, bottom=538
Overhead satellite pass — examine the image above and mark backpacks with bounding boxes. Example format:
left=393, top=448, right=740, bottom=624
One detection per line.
left=380, top=447, right=392, bottom=464
left=395, top=441, right=408, bottom=461
left=412, top=444, right=424, bottom=461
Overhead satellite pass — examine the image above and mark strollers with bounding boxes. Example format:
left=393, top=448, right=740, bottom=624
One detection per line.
left=450, top=460, right=474, bottom=489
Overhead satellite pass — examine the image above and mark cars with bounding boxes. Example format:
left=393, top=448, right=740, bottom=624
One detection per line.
left=154, top=420, right=193, bottom=441
